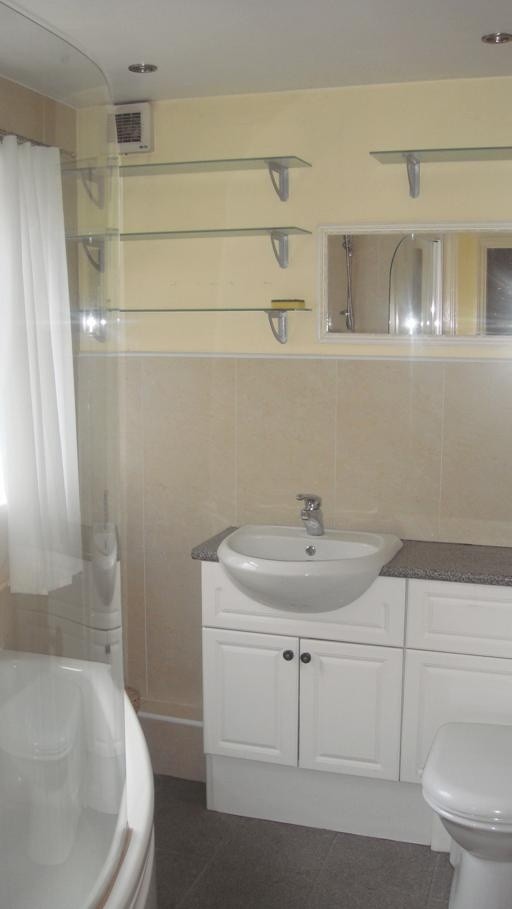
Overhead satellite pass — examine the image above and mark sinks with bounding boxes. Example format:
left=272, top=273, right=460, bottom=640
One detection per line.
left=216, top=524, right=405, bottom=615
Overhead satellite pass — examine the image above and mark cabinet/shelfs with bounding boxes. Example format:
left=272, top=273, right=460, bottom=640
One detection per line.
left=61, top=154, right=313, bottom=341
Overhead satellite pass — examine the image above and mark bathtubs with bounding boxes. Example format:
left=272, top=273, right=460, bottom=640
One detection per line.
left=0, top=646, right=162, bottom=907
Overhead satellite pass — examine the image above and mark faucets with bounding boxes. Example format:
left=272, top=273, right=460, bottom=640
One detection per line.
left=296, top=493, right=325, bottom=536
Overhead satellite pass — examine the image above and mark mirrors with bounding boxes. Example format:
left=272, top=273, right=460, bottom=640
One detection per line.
left=323, top=224, right=512, bottom=352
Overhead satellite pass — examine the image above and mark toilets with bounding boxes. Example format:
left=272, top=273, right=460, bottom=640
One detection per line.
left=421, top=721, right=512, bottom=909
